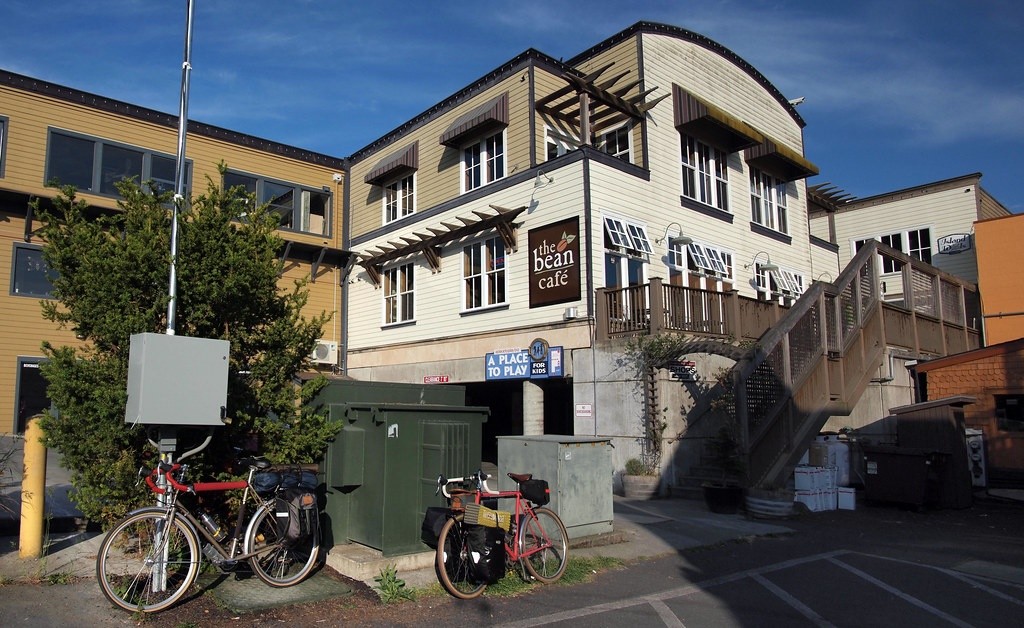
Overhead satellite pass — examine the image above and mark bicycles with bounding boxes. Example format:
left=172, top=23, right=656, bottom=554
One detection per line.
left=435, top=471, right=570, bottom=601
left=96, top=458, right=325, bottom=614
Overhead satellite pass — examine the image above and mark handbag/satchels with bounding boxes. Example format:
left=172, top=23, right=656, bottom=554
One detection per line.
left=464, top=510, right=505, bottom=586
left=519, top=479, right=551, bottom=507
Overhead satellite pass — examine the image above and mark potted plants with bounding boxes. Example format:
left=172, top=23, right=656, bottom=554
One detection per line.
left=621, top=458, right=661, bottom=500
left=744, top=481, right=796, bottom=519
left=700, top=424, right=744, bottom=514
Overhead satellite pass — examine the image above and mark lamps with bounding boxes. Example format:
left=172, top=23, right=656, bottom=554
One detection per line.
left=744, top=251, right=778, bottom=271
left=655, top=222, right=694, bottom=246
left=533, top=169, right=554, bottom=188
left=76, top=333, right=85, bottom=340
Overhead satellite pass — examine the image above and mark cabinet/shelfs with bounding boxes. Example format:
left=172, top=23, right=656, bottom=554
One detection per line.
left=862, top=445, right=953, bottom=512
left=889, top=395, right=977, bottom=509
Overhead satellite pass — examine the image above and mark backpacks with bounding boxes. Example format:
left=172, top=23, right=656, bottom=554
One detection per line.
left=274, top=470, right=319, bottom=552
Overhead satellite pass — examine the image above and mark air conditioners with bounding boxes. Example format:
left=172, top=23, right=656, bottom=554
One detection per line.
left=310, top=339, right=338, bottom=365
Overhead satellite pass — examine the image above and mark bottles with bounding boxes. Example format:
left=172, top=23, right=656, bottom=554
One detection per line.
left=202, top=541, right=225, bottom=562
left=200, top=513, right=226, bottom=543
